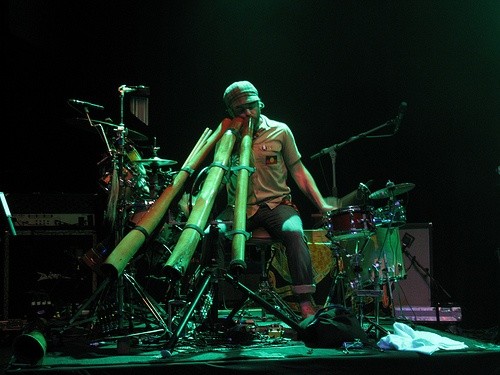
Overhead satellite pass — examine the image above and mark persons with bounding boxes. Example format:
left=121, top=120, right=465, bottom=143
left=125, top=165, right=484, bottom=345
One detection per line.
left=197, top=81, right=339, bottom=320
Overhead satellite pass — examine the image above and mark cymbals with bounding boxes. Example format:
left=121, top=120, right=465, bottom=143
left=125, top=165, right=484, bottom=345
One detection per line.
left=140, top=157, right=177, bottom=167
left=368, top=182, right=416, bottom=199
left=76, top=115, right=147, bottom=141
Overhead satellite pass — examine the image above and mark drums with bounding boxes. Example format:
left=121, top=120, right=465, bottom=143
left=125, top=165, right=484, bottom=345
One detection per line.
left=94, top=161, right=169, bottom=244
left=372, top=204, right=407, bottom=227
left=330, top=200, right=374, bottom=240
left=330, top=224, right=407, bottom=288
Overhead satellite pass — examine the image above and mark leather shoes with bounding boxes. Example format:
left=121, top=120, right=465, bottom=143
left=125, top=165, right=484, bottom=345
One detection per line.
left=301, top=297, right=317, bottom=319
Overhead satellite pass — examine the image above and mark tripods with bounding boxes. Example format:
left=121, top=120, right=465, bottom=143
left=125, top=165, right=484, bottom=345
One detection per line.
left=51, top=86, right=305, bottom=358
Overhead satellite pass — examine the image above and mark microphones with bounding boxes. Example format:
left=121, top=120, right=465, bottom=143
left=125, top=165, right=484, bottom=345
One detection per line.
left=69, top=99, right=104, bottom=110
left=393, top=102, right=407, bottom=135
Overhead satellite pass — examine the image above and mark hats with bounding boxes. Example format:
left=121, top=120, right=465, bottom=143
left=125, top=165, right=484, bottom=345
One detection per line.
left=223, top=80, right=260, bottom=107
